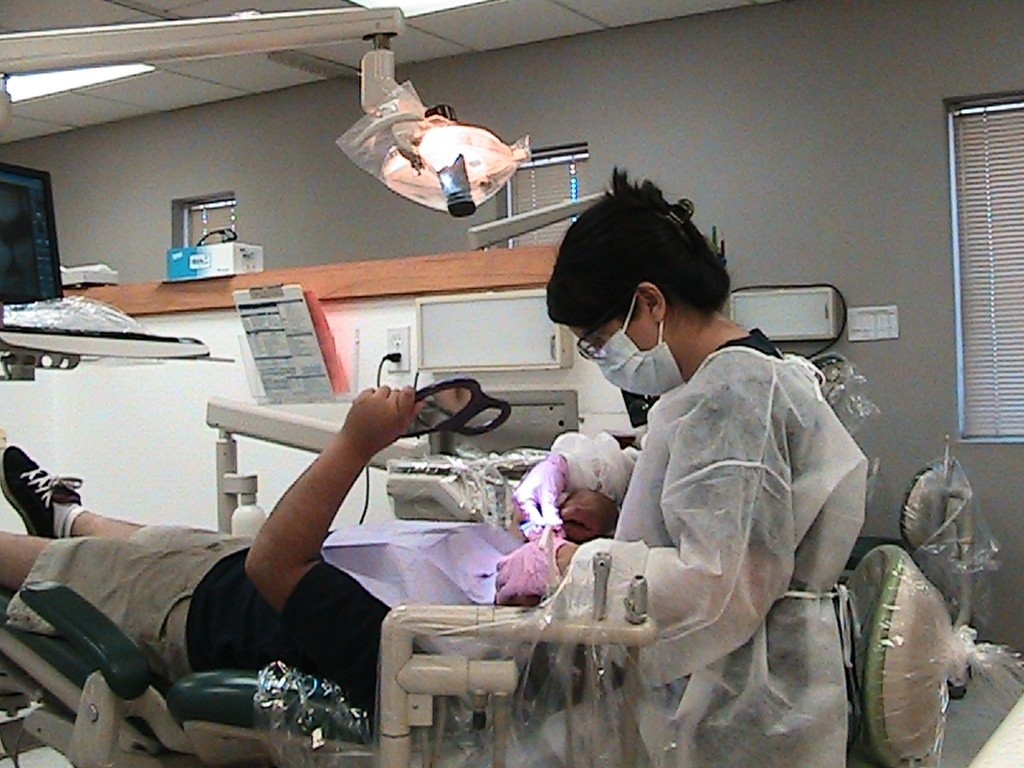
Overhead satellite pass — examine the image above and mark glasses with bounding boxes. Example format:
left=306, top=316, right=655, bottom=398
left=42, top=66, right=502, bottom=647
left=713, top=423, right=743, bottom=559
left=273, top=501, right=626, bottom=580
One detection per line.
left=577, top=311, right=621, bottom=367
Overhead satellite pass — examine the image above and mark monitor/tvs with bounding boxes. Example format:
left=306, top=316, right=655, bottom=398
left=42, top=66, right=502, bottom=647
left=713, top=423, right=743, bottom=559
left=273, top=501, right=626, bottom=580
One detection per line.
left=0, top=161, right=64, bottom=306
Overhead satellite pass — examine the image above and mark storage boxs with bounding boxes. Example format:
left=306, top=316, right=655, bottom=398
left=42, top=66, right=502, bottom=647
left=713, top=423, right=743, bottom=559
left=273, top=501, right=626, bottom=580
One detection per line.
left=165, top=243, right=264, bottom=280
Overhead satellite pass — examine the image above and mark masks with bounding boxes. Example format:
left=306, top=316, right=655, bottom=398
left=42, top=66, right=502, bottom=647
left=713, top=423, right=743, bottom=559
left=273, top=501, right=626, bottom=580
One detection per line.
left=593, top=292, right=683, bottom=396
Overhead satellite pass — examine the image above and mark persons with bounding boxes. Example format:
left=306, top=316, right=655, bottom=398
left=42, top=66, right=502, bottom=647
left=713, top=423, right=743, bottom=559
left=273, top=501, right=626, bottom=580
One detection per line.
left=0, top=385, right=623, bottom=700
left=428, top=166, right=870, bottom=768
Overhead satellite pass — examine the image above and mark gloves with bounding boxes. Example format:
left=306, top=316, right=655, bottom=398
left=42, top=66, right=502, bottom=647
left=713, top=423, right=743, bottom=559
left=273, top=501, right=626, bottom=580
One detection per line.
left=493, top=535, right=572, bottom=604
left=511, top=453, right=570, bottom=526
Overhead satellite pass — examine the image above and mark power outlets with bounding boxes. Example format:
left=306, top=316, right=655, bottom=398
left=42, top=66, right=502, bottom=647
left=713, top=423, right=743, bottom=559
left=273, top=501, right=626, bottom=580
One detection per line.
left=386, top=325, right=412, bottom=372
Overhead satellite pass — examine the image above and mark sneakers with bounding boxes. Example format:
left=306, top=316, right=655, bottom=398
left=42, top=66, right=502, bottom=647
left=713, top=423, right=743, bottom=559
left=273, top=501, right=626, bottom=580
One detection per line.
left=0, top=445, right=82, bottom=538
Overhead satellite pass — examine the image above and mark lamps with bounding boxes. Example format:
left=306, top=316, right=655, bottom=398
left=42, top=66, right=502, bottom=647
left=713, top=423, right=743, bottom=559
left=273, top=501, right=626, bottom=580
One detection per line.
left=380, top=120, right=514, bottom=216
